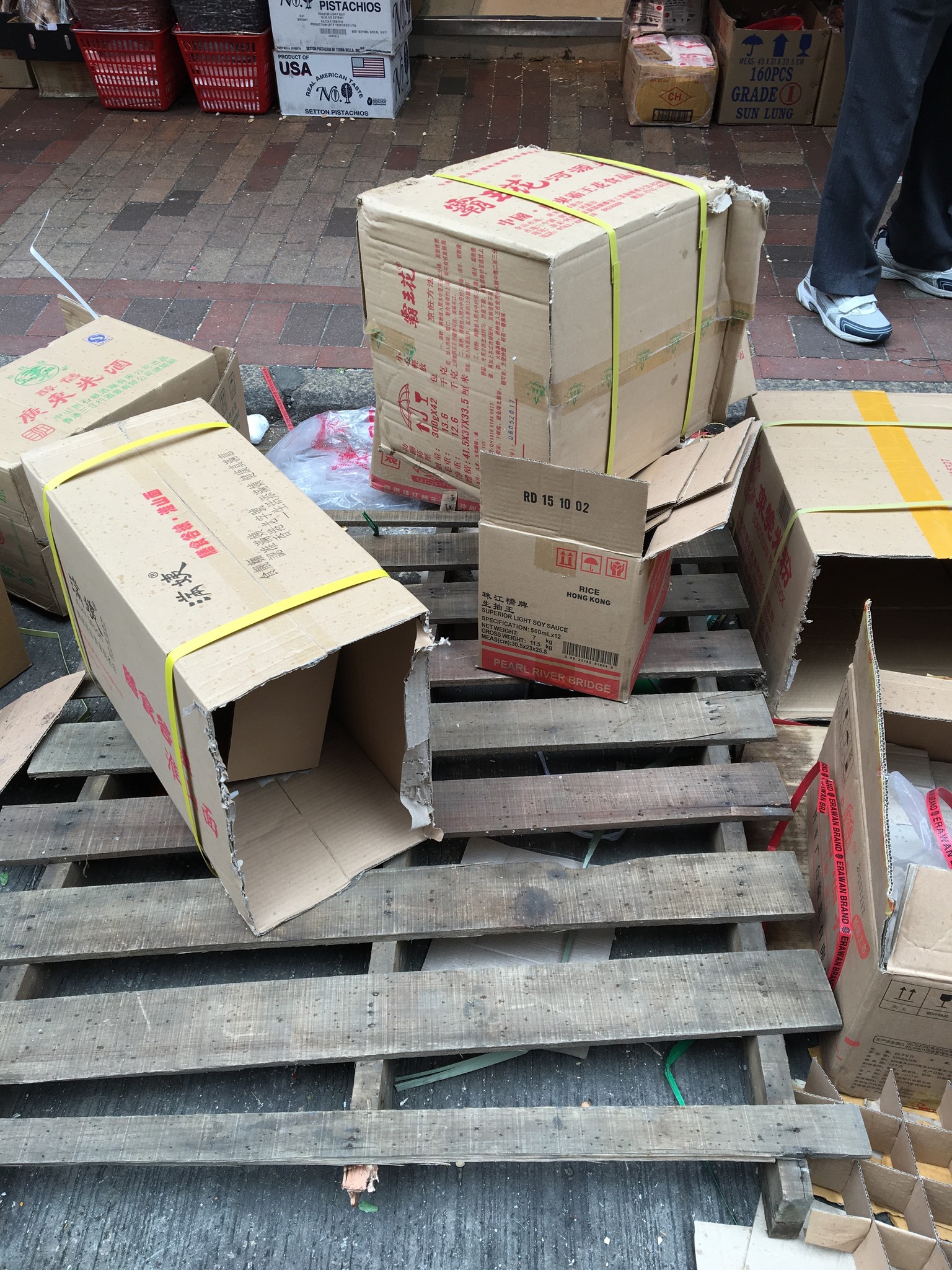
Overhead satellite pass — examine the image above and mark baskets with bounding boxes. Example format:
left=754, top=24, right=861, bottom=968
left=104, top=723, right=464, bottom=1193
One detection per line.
left=172, top=21, right=274, bottom=114
left=69, top=18, right=185, bottom=111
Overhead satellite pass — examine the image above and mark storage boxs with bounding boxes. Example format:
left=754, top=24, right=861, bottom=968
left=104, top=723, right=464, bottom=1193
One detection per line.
left=0, top=0, right=952, bottom=1270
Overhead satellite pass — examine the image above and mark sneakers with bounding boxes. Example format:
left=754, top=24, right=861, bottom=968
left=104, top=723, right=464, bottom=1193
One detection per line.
left=796, top=265, right=893, bottom=344
left=871, top=225, right=951, bottom=299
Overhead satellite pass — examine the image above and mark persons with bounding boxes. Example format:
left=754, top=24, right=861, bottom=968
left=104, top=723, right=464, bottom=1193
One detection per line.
left=795, top=0, right=952, bottom=345
